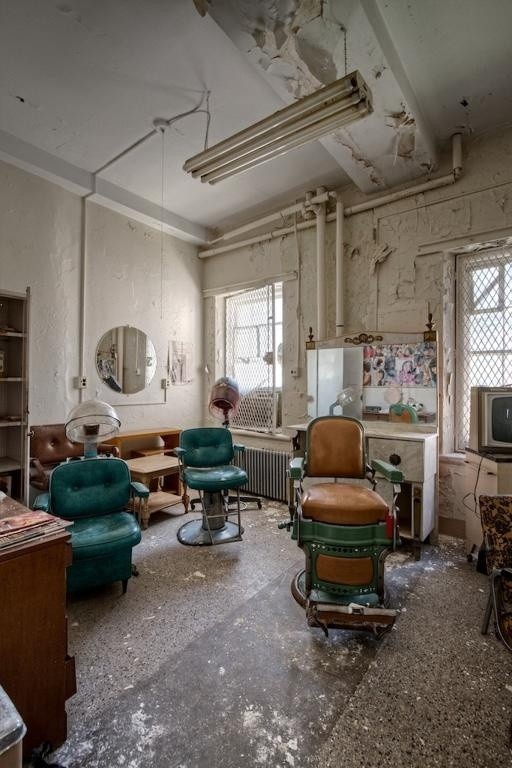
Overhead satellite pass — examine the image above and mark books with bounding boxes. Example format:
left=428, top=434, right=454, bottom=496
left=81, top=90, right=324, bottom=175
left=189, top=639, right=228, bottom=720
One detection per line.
left=0, top=507, right=73, bottom=550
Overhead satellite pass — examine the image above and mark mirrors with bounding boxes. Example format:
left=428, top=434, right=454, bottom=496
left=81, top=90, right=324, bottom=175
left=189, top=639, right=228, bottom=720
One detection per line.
left=95, top=325, right=157, bottom=395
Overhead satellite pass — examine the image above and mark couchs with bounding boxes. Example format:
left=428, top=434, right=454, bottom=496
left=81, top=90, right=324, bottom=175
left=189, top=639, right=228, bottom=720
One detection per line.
left=30, top=424, right=121, bottom=506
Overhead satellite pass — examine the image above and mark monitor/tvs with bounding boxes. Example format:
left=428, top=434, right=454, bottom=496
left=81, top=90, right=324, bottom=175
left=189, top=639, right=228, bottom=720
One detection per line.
left=482, top=391, right=512, bottom=448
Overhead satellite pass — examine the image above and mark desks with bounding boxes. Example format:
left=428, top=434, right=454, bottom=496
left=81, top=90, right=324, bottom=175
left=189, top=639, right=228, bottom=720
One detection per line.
left=463, top=446, right=512, bottom=562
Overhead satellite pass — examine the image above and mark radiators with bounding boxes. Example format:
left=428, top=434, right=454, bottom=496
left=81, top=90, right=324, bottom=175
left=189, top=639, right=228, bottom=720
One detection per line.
left=229, top=445, right=293, bottom=502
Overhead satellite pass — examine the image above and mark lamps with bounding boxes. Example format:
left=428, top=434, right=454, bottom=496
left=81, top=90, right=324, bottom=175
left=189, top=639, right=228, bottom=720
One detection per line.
left=183, top=70, right=376, bottom=185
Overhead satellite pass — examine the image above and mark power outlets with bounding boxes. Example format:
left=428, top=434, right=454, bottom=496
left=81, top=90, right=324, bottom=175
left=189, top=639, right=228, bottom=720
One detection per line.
left=290, top=369, right=300, bottom=376
left=163, top=378, right=171, bottom=390
left=78, top=377, right=88, bottom=389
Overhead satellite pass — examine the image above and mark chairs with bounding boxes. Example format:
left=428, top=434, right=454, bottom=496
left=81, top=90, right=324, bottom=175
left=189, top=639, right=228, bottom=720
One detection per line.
left=177, top=428, right=249, bottom=547
left=276, top=416, right=404, bottom=639
left=479, top=495, right=512, bottom=651
left=32, top=456, right=150, bottom=604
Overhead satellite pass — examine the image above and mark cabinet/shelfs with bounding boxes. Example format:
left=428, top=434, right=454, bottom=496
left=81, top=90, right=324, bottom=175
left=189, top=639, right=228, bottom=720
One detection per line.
left=0, top=491, right=77, bottom=763
left=285, top=423, right=439, bottom=561
left=0, top=285, right=32, bottom=508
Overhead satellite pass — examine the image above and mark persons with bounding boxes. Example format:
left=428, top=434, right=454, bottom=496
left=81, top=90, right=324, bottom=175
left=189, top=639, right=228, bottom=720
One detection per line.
left=362, top=346, right=437, bottom=388
